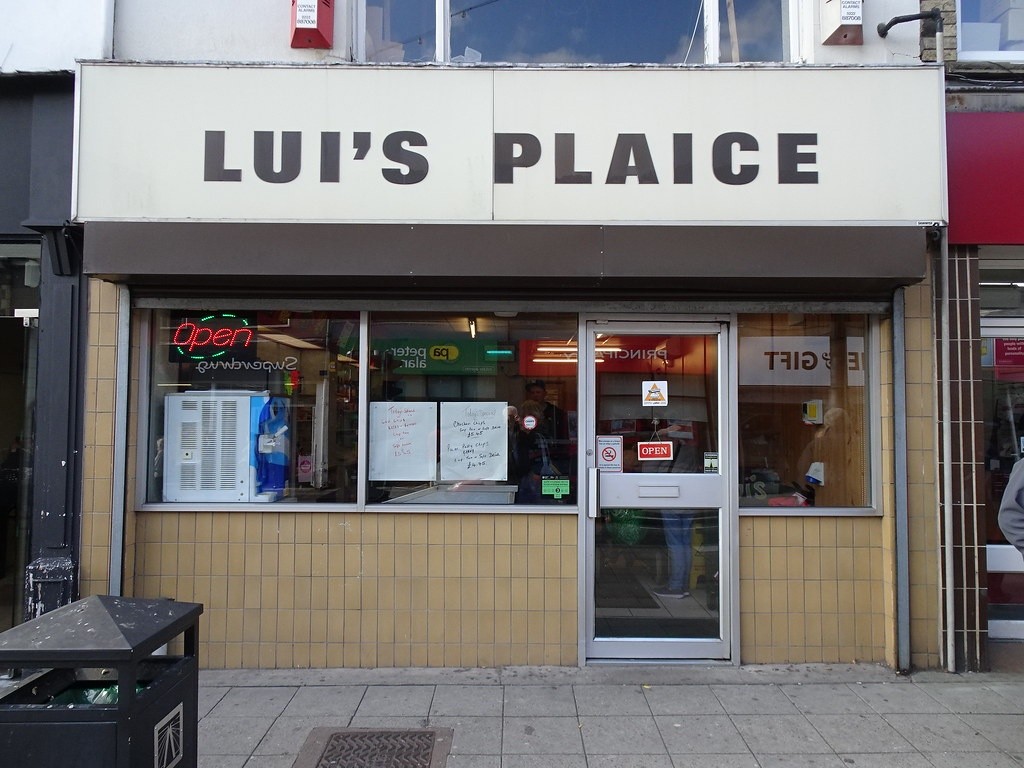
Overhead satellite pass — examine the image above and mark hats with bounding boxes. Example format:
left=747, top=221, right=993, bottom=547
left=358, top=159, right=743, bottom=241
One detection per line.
left=524, top=377, right=546, bottom=393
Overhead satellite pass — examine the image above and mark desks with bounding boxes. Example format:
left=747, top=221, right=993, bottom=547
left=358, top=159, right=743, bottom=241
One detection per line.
left=380, top=485, right=519, bottom=504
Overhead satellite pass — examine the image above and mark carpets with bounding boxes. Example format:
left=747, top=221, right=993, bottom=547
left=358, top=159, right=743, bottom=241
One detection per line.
left=596, top=543, right=668, bottom=609
left=596, top=616, right=719, bottom=637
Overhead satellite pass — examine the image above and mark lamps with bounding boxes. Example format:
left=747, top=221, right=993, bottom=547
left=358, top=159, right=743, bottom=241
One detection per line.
left=537, top=346, right=622, bottom=352
left=469, top=316, right=477, bottom=339
left=533, top=358, right=605, bottom=363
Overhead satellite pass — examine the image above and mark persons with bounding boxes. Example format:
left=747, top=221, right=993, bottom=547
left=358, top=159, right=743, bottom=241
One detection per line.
left=152, top=439, right=164, bottom=502
left=508, top=399, right=561, bottom=505
left=997, top=457, right=1024, bottom=557
left=642, top=421, right=698, bottom=597
left=525, top=381, right=569, bottom=497
left=795, top=407, right=851, bottom=489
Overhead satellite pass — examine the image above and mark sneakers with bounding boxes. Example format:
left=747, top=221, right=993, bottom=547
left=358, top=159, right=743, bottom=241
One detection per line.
left=652, top=585, right=684, bottom=599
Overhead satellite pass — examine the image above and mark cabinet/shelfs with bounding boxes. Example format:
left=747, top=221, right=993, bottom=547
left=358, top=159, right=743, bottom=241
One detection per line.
left=295, top=354, right=359, bottom=432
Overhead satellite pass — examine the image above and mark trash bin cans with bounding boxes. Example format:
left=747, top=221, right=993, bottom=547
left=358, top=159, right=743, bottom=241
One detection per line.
left=0, top=593, right=204, bottom=767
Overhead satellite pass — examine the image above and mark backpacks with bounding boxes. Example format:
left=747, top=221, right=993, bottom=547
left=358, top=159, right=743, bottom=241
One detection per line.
left=526, top=432, right=569, bottom=504
